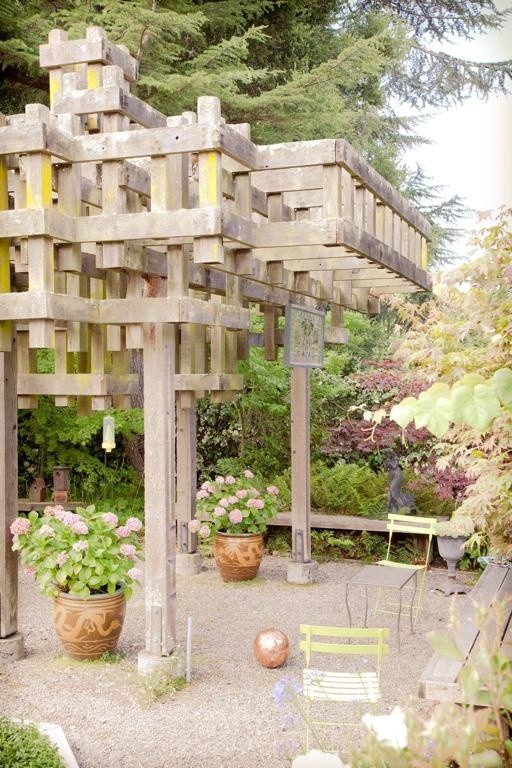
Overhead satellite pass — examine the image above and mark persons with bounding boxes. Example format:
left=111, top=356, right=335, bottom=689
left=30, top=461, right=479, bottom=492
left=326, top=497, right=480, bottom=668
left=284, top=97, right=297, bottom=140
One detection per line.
left=382, top=449, right=404, bottom=513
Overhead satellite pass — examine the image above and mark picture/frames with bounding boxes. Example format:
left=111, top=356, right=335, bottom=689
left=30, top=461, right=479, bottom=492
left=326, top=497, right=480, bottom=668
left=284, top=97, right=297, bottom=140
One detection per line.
left=214, top=527, right=263, bottom=581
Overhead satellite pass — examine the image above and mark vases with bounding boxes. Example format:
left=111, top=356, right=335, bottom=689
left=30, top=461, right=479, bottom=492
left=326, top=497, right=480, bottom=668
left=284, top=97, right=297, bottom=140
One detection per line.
left=54, top=580, right=129, bottom=661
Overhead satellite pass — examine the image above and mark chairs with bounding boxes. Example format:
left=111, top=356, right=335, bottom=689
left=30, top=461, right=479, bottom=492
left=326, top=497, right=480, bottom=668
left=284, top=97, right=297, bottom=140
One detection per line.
left=375, top=513, right=438, bottom=622
left=297, top=626, right=390, bottom=758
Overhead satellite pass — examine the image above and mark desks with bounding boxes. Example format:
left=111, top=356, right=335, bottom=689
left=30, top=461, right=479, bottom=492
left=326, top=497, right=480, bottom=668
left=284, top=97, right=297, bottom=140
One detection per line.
left=344, top=566, right=417, bottom=653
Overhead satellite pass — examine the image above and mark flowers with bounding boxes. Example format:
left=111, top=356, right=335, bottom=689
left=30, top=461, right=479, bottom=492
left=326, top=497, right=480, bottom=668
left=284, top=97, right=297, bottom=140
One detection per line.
left=9, top=503, right=143, bottom=599
left=187, top=469, right=282, bottom=539
